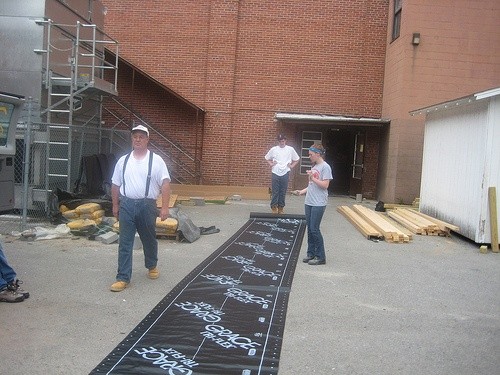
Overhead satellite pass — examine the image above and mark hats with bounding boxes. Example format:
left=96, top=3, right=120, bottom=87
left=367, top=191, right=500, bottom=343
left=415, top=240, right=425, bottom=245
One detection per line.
left=132, top=125, right=149, bottom=139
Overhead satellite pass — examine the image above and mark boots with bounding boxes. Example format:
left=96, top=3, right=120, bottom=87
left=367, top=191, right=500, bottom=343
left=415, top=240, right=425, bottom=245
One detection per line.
left=271, top=206, right=278, bottom=214
left=278, top=205, right=283, bottom=214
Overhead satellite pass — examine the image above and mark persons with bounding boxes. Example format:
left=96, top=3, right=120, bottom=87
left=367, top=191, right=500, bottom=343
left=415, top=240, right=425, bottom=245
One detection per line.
left=0, top=239, right=29, bottom=303
left=110, top=125, right=170, bottom=292
left=290, top=141, right=332, bottom=265
left=265, top=133, right=300, bottom=214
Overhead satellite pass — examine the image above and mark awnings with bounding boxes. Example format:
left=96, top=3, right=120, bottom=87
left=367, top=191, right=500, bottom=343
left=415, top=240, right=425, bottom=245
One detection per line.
left=273, top=113, right=390, bottom=126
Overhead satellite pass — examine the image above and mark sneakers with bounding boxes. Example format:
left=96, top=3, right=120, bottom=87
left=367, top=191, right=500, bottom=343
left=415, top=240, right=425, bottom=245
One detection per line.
left=0, top=286, right=24, bottom=303
left=309, top=256, right=326, bottom=265
left=8, top=279, right=29, bottom=297
left=304, top=255, right=313, bottom=263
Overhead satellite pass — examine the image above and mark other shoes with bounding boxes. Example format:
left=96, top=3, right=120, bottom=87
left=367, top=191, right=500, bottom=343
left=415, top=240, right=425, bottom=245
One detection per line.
left=149, top=267, right=159, bottom=279
left=111, top=280, right=129, bottom=291
